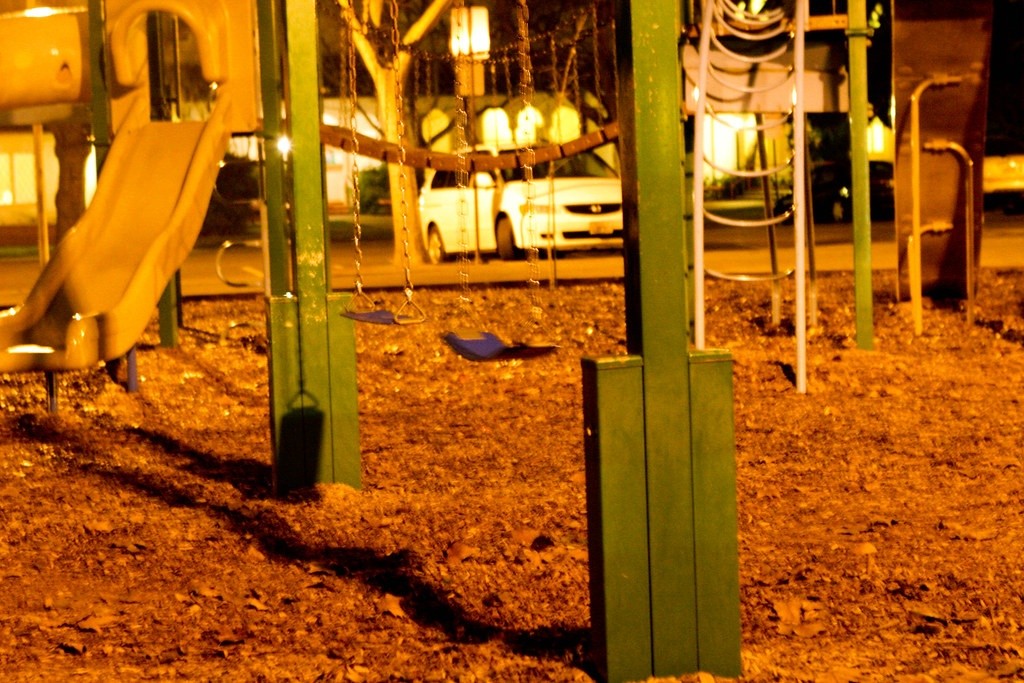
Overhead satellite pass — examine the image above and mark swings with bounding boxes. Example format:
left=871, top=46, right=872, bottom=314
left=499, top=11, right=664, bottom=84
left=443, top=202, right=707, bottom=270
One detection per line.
left=341, top=1, right=561, bottom=363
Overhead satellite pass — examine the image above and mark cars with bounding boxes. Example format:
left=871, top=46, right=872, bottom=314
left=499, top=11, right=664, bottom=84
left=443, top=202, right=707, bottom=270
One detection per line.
left=418, top=140, right=624, bottom=266
left=772, top=158, right=895, bottom=226
